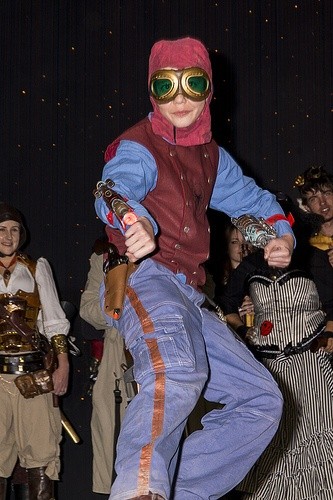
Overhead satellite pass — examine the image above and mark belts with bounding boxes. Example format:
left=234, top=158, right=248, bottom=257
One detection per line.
left=0, top=352, right=45, bottom=374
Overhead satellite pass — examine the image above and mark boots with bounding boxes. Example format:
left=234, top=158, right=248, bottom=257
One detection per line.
left=0, top=477, right=7, bottom=500
left=27, top=465, right=55, bottom=500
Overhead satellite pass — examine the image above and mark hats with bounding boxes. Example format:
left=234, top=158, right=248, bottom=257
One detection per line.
left=148, top=38, right=213, bottom=146
left=0, top=202, right=27, bottom=249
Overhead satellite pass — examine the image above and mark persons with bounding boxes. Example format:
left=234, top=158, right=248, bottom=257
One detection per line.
left=220, top=220, right=259, bottom=285
left=216, top=192, right=333, bottom=500
left=78, top=238, right=142, bottom=500
left=93, top=38, right=297, bottom=500
left=298, top=177, right=333, bottom=267
left=0, top=206, right=69, bottom=500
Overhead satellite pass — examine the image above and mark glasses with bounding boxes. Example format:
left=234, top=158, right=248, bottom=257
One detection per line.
left=150, top=66, right=212, bottom=104
left=290, top=164, right=327, bottom=191
